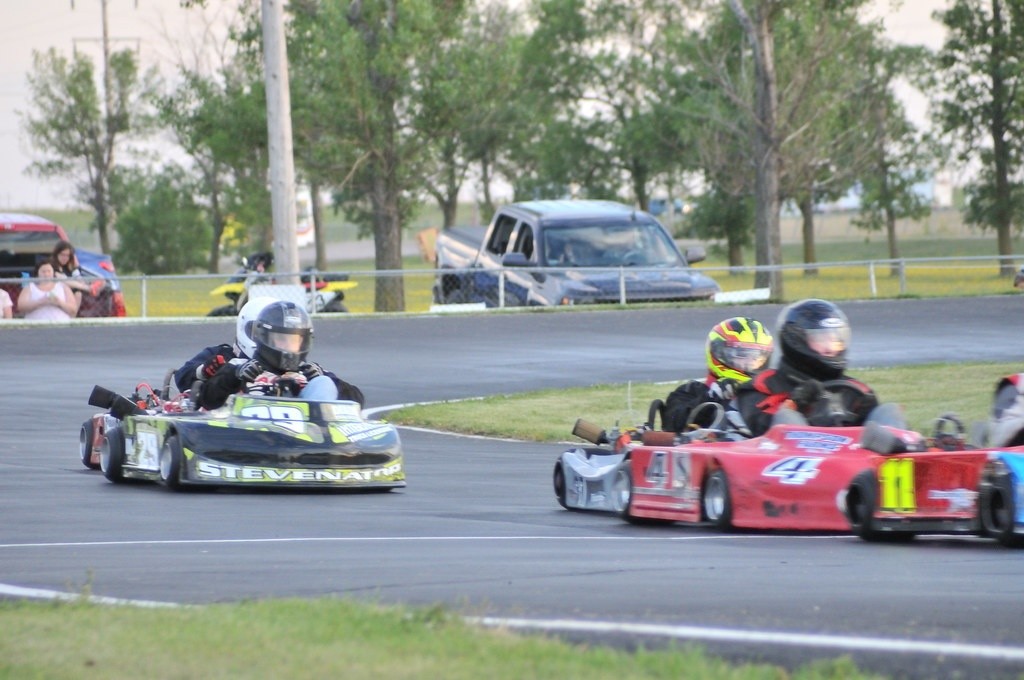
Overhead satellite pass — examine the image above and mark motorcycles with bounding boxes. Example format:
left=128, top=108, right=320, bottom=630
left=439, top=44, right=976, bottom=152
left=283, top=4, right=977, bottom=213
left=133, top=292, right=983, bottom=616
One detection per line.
left=207, top=251, right=359, bottom=316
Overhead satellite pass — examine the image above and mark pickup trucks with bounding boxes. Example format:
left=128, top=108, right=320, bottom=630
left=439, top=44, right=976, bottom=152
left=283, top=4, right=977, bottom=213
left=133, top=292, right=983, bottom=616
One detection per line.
left=0, top=213, right=114, bottom=321
left=432, top=198, right=722, bottom=308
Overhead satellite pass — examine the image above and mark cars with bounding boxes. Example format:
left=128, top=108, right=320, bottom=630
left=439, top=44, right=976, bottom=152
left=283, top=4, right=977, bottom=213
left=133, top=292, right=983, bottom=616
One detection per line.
left=73, top=247, right=119, bottom=290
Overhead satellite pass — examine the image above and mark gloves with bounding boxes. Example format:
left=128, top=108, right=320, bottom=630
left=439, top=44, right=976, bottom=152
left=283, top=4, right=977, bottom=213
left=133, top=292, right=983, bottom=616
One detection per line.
left=707, top=375, right=741, bottom=403
left=201, top=354, right=228, bottom=379
left=234, top=358, right=265, bottom=383
left=787, top=379, right=826, bottom=414
left=297, top=361, right=324, bottom=382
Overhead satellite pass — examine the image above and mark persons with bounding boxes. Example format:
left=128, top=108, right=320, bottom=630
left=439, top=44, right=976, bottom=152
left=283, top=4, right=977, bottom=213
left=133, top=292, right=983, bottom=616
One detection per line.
left=166, top=294, right=367, bottom=412
left=990, top=373, right=1024, bottom=448
left=0, top=240, right=106, bottom=318
left=243, top=252, right=274, bottom=278
left=662, top=298, right=878, bottom=438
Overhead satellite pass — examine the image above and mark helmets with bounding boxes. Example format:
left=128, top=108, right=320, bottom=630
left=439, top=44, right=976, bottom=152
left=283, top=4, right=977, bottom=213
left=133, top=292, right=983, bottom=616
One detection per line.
left=775, top=299, right=852, bottom=381
left=232, top=295, right=315, bottom=375
left=704, top=316, right=774, bottom=392
